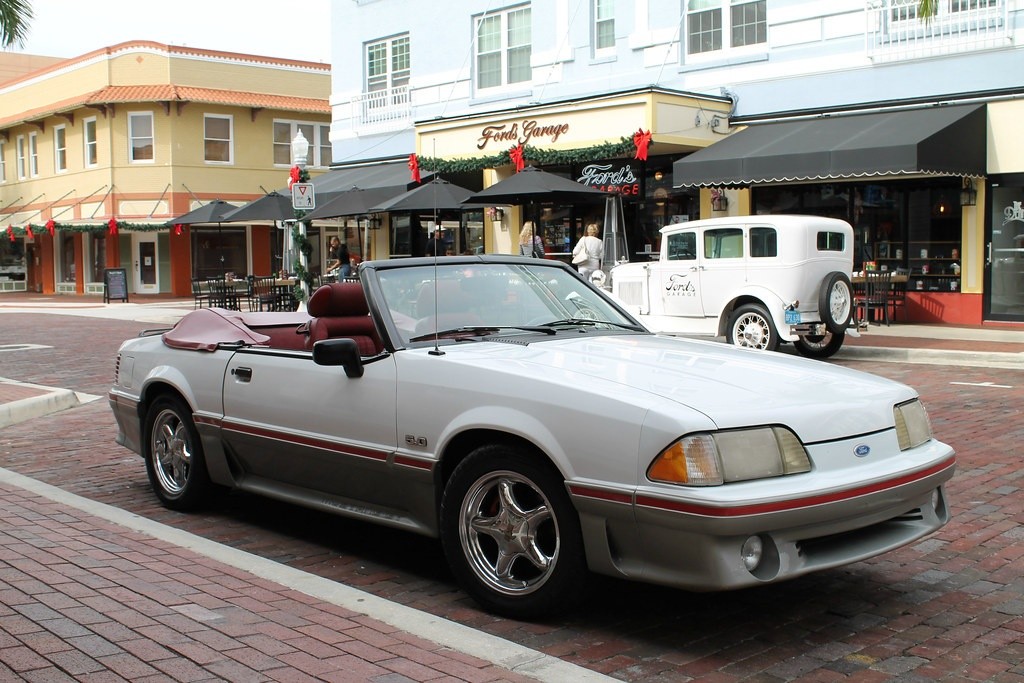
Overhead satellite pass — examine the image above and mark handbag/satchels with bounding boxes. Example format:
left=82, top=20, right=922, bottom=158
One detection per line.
left=572, top=236, right=589, bottom=265
left=532, top=236, right=543, bottom=258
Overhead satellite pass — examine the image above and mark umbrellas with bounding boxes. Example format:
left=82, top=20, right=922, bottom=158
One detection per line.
left=460, top=164, right=610, bottom=251
left=804, top=192, right=881, bottom=208
left=771, top=195, right=803, bottom=210
left=297, top=184, right=388, bottom=263
left=540, top=208, right=571, bottom=222
left=163, top=197, right=239, bottom=290
left=219, top=189, right=307, bottom=311
left=369, top=175, right=512, bottom=239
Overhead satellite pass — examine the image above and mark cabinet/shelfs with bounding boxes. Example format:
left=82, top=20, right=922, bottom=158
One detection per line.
left=873, top=241, right=961, bottom=293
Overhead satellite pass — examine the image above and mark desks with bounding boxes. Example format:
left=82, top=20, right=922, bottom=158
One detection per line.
left=851, top=274, right=907, bottom=322
left=254, top=279, right=300, bottom=310
left=206, top=280, right=246, bottom=308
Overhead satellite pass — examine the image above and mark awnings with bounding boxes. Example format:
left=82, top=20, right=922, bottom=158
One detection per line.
left=673, top=103, right=987, bottom=190
left=276, top=162, right=453, bottom=214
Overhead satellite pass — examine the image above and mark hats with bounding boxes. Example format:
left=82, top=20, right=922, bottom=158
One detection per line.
left=436, top=225, right=447, bottom=231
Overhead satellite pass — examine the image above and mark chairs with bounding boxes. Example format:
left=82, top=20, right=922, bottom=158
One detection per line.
left=303, top=282, right=383, bottom=357
left=879, top=266, right=913, bottom=322
left=853, top=269, right=892, bottom=327
left=414, top=277, right=481, bottom=334
left=190, top=272, right=360, bottom=313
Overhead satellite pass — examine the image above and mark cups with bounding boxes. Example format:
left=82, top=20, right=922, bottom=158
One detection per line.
left=920, top=249, right=927, bottom=258
left=880, top=265, right=888, bottom=271
left=953, top=265, right=960, bottom=275
left=915, top=280, right=924, bottom=290
left=951, top=281, right=958, bottom=291
left=853, top=271, right=896, bottom=277
left=896, top=249, right=903, bottom=259
left=952, top=249, right=958, bottom=259
left=922, top=264, right=929, bottom=275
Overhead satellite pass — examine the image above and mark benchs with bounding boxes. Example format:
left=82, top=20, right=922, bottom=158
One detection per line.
left=250, top=325, right=305, bottom=350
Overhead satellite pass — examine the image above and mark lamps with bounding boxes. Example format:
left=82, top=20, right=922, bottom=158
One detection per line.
left=711, top=187, right=727, bottom=211
left=489, top=207, right=504, bottom=221
left=367, top=214, right=383, bottom=230
left=960, top=177, right=977, bottom=205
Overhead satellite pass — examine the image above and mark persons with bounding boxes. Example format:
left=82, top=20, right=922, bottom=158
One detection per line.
left=573, top=224, right=603, bottom=280
left=327, top=237, right=351, bottom=283
left=519, top=221, right=544, bottom=258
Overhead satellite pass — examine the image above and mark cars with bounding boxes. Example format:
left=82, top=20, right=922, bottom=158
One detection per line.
left=588, top=215, right=854, bottom=360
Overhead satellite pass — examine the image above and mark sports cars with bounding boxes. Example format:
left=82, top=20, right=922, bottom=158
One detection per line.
left=109, top=254, right=956, bottom=625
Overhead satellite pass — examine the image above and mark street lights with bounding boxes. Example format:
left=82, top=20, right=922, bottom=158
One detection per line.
left=292, top=128, right=309, bottom=314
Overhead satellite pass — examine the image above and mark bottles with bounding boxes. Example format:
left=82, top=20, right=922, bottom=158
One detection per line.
left=564, top=238, right=570, bottom=252
